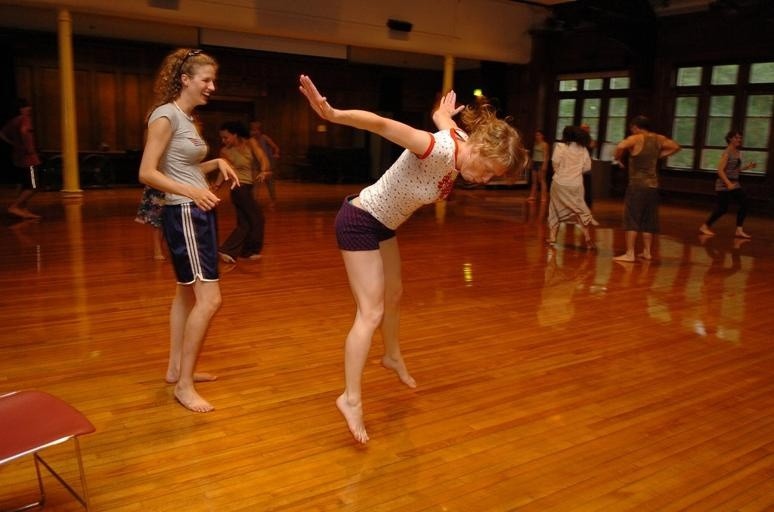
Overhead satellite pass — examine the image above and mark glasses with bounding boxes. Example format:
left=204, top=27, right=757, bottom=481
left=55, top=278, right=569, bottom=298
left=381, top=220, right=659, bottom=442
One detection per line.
left=181, top=49, right=205, bottom=68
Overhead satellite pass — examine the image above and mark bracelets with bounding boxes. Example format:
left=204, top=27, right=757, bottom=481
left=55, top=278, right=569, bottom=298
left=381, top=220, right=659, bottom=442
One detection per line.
left=260, top=170, right=267, bottom=172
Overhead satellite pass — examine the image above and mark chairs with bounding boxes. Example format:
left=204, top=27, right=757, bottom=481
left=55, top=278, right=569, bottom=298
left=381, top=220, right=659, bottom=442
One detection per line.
left=0, top=390, right=97, bottom=512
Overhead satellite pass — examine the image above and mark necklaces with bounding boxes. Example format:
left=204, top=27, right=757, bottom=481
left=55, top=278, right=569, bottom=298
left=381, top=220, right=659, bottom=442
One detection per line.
left=172, top=100, right=194, bottom=122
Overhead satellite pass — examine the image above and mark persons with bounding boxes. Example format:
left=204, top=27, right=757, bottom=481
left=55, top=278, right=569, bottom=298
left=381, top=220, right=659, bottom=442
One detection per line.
left=525, top=130, right=549, bottom=202
left=612, top=113, right=679, bottom=262
left=211, top=121, right=275, bottom=263
left=248, top=121, right=281, bottom=195
left=299, top=74, right=531, bottom=444
left=0, top=97, right=44, bottom=219
left=139, top=47, right=241, bottom=413
left=545, top=123, right=600, bottom=250
left=133, top=185, right=167, bottom=261
left=698, top=127, right=757, bottom=238
